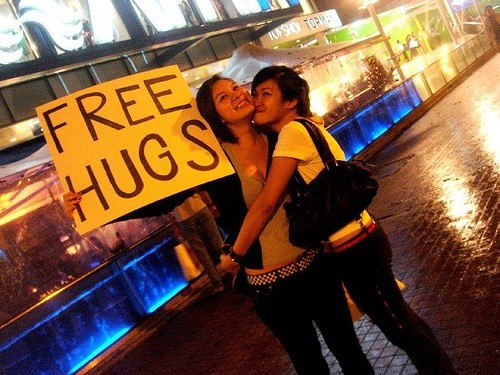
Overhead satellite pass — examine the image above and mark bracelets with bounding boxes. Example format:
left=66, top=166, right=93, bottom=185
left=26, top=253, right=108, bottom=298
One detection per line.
left=227, top=247, right=245, bottom=263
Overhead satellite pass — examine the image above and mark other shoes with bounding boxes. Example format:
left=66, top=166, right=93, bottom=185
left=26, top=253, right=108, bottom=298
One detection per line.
left=212, top=287, right=224, bottom=294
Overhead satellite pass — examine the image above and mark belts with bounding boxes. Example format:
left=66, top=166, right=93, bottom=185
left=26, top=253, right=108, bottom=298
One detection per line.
left=320, top=217, right=376, bottom=254
left=244, top=245, right=319, bottom=286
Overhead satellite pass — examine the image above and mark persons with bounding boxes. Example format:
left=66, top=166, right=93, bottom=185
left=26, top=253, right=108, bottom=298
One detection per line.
left=216, top=65, right=458, bottom=375
left=484, top=5, right=500, bottom=53
left=396, top=23, right=442, bottom=61
left=63, top=74, right=376, bottom=375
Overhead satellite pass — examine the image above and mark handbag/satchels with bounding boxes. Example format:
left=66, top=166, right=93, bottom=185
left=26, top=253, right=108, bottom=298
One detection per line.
left=283, top=119, right=379, bottom=250
